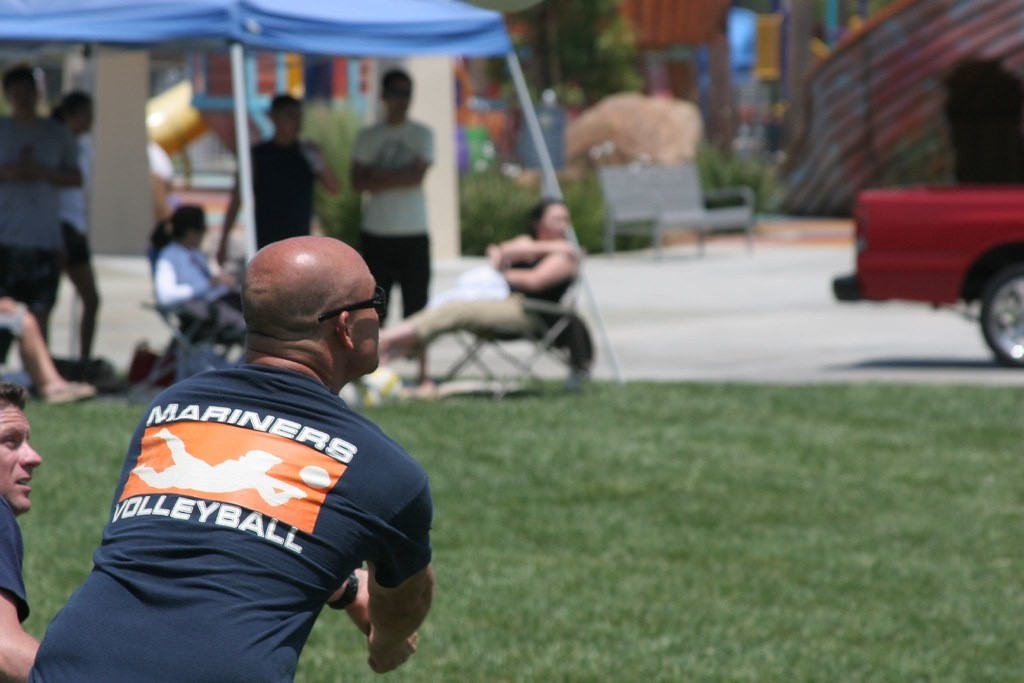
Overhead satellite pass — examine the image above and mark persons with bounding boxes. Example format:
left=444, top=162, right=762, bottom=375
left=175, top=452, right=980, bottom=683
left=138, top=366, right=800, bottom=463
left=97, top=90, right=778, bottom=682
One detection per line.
left=27, top=236, right=433, bottom=683
left=0, top=66, right=115, bottom=405
left=0, top=382, right=42, bottom=683
left=149, top=68, right=436, bottom=407
left=374, top=197, right=581, bottom=364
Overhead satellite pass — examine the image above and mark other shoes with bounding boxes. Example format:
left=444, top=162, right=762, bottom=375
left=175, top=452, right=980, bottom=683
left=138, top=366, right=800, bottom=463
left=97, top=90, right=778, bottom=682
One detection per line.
left=40, top=382, right=97, bottom=405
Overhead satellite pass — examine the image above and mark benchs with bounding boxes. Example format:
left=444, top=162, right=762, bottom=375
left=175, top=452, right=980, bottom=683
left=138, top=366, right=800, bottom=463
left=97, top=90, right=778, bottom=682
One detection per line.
left=596, top=162, right=758, bottom=259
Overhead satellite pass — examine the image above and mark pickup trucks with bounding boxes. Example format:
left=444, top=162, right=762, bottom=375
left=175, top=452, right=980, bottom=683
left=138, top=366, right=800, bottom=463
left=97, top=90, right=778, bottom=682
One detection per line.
left=834, top=190, right=1023, bottom=371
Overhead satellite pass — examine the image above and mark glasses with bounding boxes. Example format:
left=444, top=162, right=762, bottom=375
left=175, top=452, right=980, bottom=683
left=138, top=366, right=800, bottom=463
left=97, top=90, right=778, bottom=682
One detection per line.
left=318, top=286, right=386, bottom=322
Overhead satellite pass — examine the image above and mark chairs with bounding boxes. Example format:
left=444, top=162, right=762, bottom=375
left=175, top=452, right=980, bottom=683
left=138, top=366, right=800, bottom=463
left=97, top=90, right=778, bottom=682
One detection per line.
left=127, top=248, right=246, bottom=407
left=442, top=246, right=587, bottom=401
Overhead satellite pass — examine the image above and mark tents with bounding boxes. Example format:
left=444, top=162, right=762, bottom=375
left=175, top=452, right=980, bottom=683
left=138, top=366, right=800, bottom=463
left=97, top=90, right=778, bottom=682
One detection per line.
left=0, top=0, right=622, bottom=384
